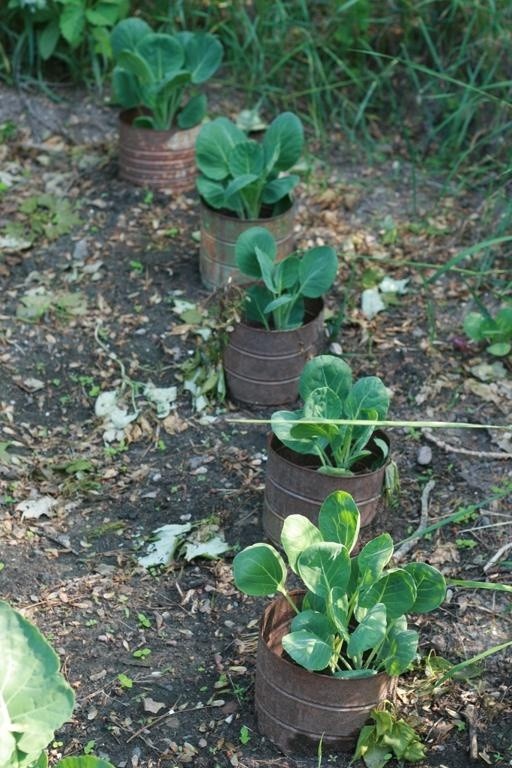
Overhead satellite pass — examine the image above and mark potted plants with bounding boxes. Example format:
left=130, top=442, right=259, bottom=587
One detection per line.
left=221, top=225, right=338, bottom=405
left=262, top=354, right=392, bottom=550
left=233, top=489, right=447, bottom=757
left=110, top=17, right=223, bottom=193
left=194, top=110, right=305, bottom=291
